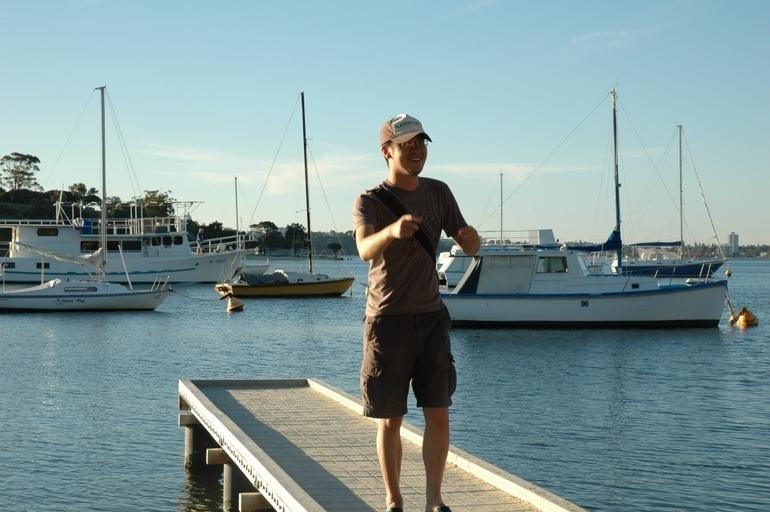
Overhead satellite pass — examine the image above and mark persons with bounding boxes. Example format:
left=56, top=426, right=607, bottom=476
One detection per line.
left=196, top=228, right=204, bottom=256
left=352, top=114, right=481, bottom=512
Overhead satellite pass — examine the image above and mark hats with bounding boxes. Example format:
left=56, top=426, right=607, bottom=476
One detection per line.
left=380, top=112, right=432, bottom=146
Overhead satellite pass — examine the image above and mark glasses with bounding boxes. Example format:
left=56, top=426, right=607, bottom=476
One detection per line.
left=386, top=139, right=429, bottom=150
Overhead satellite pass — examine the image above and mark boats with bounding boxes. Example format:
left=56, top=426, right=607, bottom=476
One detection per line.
left=0, top=202, right=256, bottom=286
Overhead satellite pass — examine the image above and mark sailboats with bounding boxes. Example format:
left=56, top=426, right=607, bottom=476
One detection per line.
left=215, top=91, right=355, bottom=299
left=435, top=251, right=729, bottom=329
left=0, top=84, right=175, bottom=313
